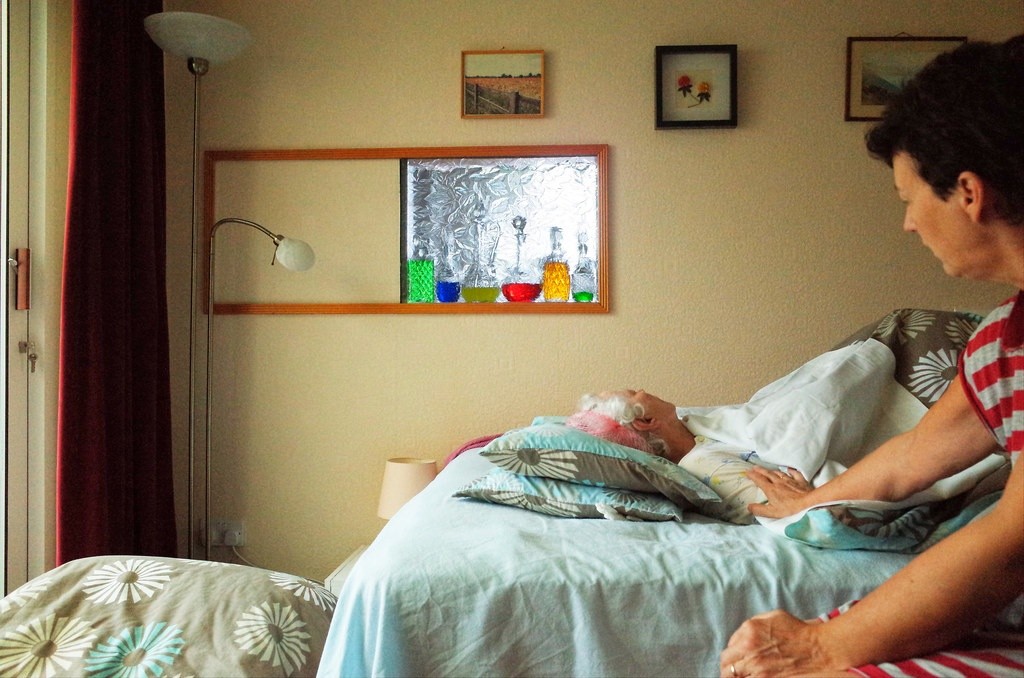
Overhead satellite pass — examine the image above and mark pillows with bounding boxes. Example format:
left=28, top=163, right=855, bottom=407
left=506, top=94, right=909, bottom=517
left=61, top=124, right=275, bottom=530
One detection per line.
left=479, top=416, right=722, bottom=511
left=451, top=466, right=684, bottom=522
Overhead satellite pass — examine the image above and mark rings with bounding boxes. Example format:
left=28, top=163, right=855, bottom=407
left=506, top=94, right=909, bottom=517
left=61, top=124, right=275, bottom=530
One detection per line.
left=731, top=664, right=737, bottom=676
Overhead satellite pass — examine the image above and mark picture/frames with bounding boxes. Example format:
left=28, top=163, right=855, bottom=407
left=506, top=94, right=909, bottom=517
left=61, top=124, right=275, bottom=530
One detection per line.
left=655, top=44, right=738, bottom=129
left=460, top=49, right=545, bottom=119
left=844, top=36, right=967, bottom=121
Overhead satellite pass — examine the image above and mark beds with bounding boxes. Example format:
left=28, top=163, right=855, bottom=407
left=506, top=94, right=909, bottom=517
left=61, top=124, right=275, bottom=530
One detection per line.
left=315, top=309, right=1012, bottom=678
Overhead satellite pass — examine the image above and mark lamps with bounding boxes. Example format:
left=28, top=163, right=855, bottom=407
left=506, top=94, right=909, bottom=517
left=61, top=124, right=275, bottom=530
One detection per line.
left=142, top=11, right=252, bottom=559
left=378, top=457, right=436, bottom=520
left=205, top=218, right=314, bottom=561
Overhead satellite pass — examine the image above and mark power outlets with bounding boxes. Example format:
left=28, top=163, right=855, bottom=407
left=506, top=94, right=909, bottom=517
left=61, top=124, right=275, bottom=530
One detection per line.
left=201, top=519, right=245, bottom=546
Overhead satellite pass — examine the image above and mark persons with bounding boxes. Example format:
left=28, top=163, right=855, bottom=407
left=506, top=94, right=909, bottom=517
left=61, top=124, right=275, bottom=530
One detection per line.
left=718, top=32, right=1023, bottom=678
left=569, top=387, right=768, bottom=526
